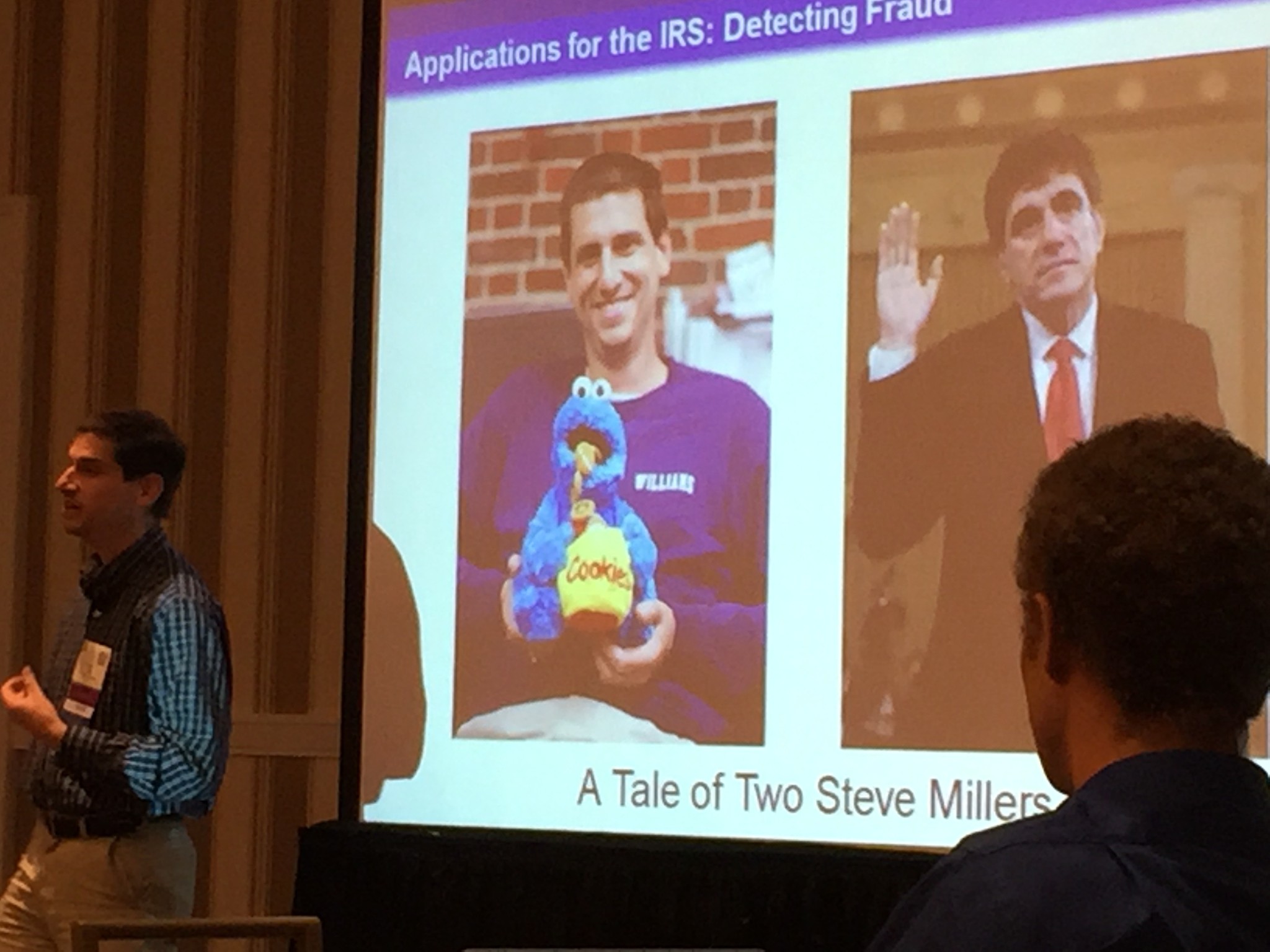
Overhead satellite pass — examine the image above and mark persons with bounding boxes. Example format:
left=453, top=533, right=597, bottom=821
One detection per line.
left=0, top=404, right=233, bottom=952
left=851, top=132, right=1227, bottom=747
left=902, top=418, right=1270, bottom=952
left=460, top=152, right=770, bottom=744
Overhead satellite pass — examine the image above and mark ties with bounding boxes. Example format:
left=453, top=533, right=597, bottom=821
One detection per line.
left=1043, top=337, right=1085, bottom=461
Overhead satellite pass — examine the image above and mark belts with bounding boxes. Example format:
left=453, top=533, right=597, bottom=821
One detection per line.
left=36, top=801, right=178, bottom=840
